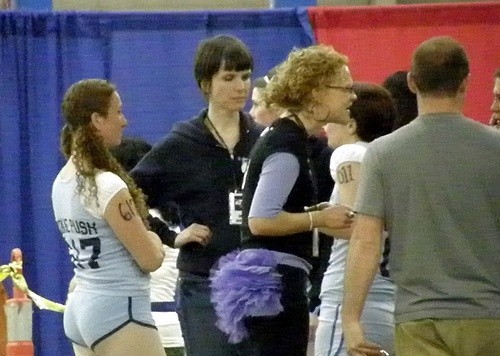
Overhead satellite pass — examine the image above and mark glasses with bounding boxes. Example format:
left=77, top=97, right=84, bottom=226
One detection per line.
left=324, top=84, right=356, bottom=94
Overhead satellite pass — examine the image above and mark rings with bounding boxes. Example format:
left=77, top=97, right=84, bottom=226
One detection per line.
left=348, top=211, right=353, bottom=218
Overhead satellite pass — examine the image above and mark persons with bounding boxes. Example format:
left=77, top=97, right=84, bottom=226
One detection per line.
left=51, top=79, right=165, bottom=356
left=126, top=35, right=267, bottom=356
left=342, top=37, right=500, bottom=356
left=249, top=76, right=286, bottom=126
left=105, top=141, right=186, bottom=356
left=208, top=44, right=356, bottom=356
left=316, top=83, right=396, bottom=356
left=489, top=72, right=499, bottom=125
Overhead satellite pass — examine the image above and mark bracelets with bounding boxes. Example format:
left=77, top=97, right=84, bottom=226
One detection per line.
left=308, top=212, right=313, bottom=232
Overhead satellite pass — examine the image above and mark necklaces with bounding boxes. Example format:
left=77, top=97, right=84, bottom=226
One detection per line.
left=204, top=113, right=232, bottom=150
left=290, top=112, right=308, bottom=133
